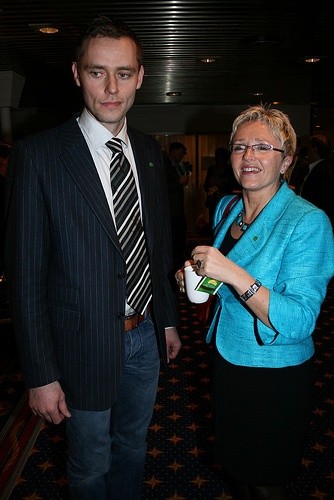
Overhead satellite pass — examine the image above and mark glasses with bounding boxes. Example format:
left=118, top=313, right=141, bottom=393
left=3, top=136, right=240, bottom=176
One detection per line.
left=228, top=144, right=285, bottom=153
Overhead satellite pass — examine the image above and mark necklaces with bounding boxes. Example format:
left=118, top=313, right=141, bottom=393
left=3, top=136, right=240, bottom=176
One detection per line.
left=236, top=207, right=251, bottom=233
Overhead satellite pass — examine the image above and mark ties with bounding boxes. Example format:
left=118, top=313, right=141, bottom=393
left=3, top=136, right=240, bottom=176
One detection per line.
left=105, top=138, right=152, bottom=315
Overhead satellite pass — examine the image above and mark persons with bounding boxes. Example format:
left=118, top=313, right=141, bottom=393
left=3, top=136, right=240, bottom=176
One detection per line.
left=175, top=102, right=334, bottom=500
left=0, top=16, right=182, bottom=500
left=167, top=134, right=334, bottom=258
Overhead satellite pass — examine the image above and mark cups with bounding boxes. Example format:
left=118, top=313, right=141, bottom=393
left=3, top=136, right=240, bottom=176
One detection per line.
left=185, top=265, right=209, bottom=304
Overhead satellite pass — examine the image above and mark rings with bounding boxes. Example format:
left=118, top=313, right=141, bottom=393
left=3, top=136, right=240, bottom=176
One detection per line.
left=195, top=259, right=202, bottom=269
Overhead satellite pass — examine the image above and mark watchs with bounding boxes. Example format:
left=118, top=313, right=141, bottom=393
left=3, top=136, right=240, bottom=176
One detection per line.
left=240, top=279, right=262, bottom=302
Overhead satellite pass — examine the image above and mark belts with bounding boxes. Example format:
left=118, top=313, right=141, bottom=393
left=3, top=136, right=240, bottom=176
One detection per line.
left=124, top=314, right=143, bottom=332
